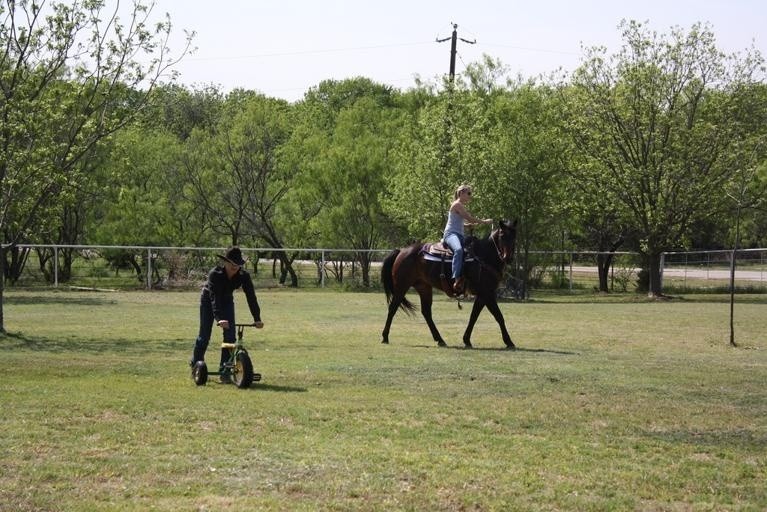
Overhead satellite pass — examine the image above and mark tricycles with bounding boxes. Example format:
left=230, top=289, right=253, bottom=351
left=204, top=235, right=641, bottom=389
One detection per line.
left=189, top=319, right=264, bottom=388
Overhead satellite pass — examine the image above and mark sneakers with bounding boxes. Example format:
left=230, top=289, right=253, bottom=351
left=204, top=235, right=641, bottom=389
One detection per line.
left=220, top=375, right=231, bottom=382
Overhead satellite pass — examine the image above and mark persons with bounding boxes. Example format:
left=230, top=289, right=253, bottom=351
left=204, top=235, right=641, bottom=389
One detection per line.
left=443, top=185, right=493, bottom=290
left=190, top=247, right=263, bottom=384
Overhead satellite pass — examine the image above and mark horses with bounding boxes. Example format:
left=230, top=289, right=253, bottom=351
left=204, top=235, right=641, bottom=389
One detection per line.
left=381, top=216, right=519, bottom=349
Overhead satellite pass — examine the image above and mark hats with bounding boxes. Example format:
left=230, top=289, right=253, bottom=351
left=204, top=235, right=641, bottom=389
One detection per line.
left=216, top=247, right=250, bottom=267
